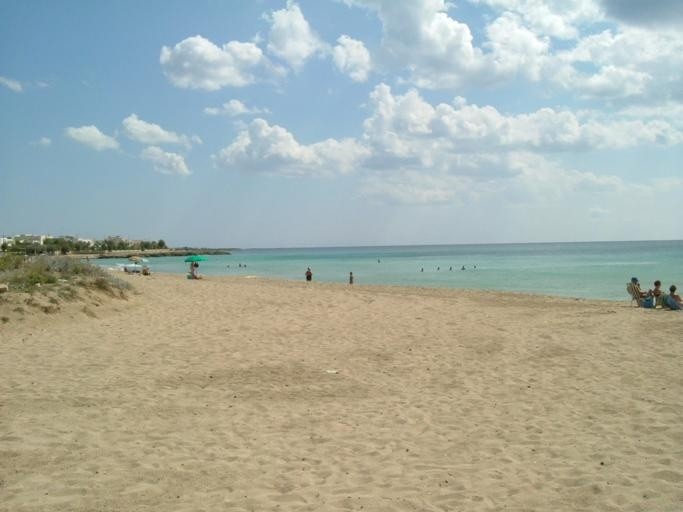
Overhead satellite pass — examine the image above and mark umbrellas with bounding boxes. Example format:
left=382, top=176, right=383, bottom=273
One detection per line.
left=185, top=256, right=208, bottom=262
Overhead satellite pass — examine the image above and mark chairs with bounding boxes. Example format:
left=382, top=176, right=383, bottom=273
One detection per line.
left=626, top=283, right=683, bottom=310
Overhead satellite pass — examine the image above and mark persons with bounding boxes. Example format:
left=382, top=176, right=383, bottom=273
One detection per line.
left=304, top=266, right=312, bottom=281
left=143, top=265, right=150, bottom=275
left=653, top=280, right=663, bottom=297
left=669, top=285, right=683, bottom=304
left=631, top=277, right=653, bottom=298
left=190, top=261, right=196, bottom=276
left=349, top=272, right=353, bottom=284
left=461, top=266, right=466, bottom=270
